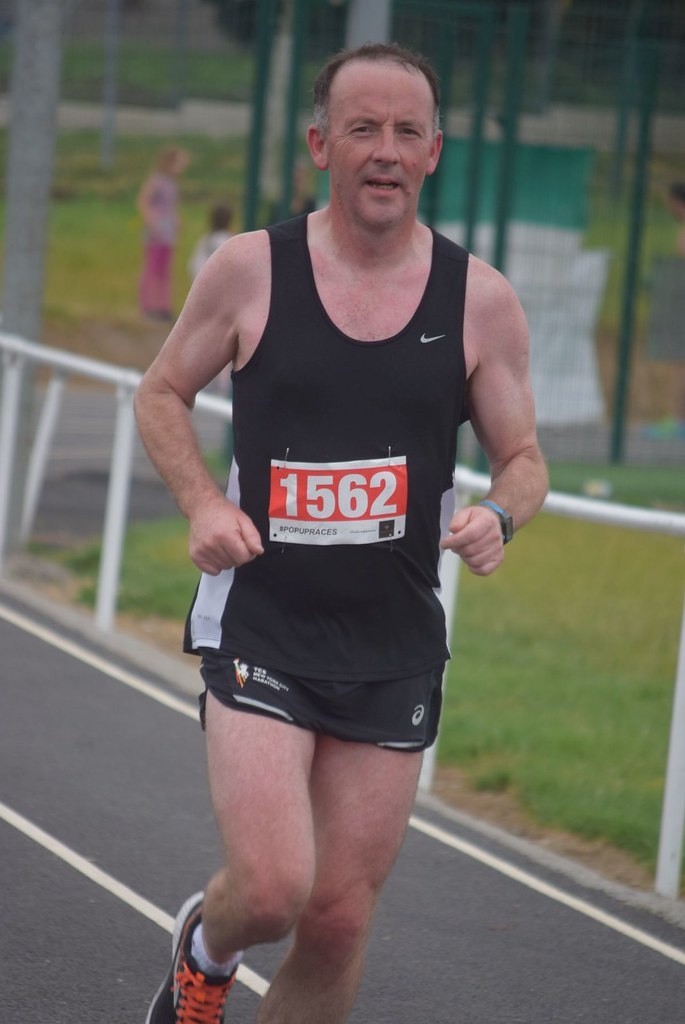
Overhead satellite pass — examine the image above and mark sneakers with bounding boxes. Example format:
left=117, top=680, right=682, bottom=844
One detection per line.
left=146, top=892, right=244, bottom=1024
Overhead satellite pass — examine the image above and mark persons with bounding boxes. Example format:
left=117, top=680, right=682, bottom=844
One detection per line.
left=132, top=42, right=549, bottom=1024
left=133, top=146, right=190, bottom=325
left=264, top=158, right=317, bottom=227
left=189, top=202, right=236, bottom=284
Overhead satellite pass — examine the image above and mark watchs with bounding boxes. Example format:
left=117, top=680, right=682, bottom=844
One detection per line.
left=476, top=500, right=513, bottom=546
